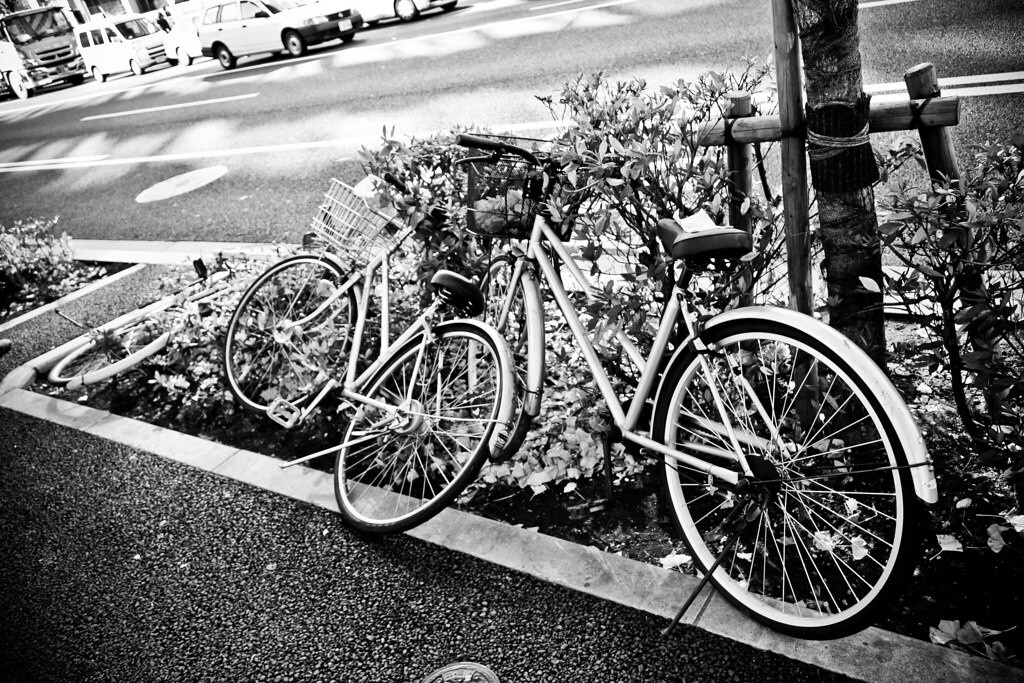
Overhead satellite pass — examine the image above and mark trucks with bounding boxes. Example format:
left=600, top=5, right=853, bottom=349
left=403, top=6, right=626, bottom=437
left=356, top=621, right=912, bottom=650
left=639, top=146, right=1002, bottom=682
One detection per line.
left=0, top=3, right=89, bottom=101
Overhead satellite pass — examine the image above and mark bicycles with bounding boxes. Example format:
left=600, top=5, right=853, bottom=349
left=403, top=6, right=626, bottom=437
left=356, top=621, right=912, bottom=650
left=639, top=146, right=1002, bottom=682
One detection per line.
left=223, top=170, right=521, bottom=536
left=454, top=131, right=939, bottom=640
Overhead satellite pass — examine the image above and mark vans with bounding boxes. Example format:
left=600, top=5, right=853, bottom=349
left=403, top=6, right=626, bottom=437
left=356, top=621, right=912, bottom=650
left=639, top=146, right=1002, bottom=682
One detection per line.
left=73, top=12, right=178, bottom=84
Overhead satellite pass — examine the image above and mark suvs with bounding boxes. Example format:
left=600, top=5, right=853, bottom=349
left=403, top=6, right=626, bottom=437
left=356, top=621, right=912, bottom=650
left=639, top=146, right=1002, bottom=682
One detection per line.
left=194, top=0, right=365, bottom=70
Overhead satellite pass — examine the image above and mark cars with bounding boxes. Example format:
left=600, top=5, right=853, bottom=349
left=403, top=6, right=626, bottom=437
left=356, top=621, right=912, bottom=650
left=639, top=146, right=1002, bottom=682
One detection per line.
left=352, top=0, right=459, bottom=27
left=164, top=9, right=204, bottom=66
